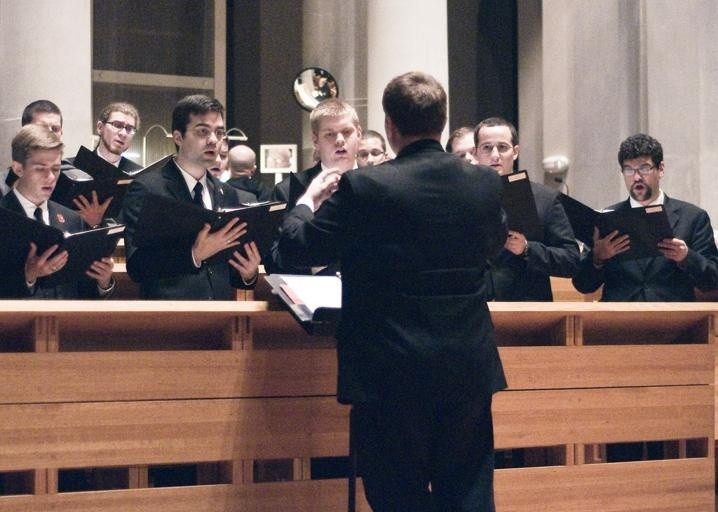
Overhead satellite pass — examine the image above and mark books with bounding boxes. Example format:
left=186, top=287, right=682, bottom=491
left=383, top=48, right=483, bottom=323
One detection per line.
left=135, top=191, right=272, bottom=268
left=8, top=218, right=127, bottom=286
left=501, top=171, right=544, bottom=232
left=48, top=170, right=130, bottom=224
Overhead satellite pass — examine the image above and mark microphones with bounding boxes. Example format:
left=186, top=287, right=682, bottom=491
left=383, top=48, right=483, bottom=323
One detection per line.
left=554, top=176, right=568, bottom=196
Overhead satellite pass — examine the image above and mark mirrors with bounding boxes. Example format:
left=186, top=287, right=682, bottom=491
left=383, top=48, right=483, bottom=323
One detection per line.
left=293, top=67, right=339, bottom=111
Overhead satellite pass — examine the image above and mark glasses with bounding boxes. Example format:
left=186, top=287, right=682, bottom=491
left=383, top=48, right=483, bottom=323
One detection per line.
left=183, top=127, right=227, bottom=140
left=622, top=164, right=657, bottom=176
left=354, top=149, right=384, bottom=160
left=102, top=122, right=137, bottom=136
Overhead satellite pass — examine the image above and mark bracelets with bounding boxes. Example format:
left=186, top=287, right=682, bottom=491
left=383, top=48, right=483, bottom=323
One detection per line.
left=523, top=238, right=530, bottom=256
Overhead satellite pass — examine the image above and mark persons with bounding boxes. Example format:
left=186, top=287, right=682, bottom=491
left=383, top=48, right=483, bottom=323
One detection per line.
left=249, top=99, right=374, bottom=277
left=475, top=117, right=580, bottom=304
left=444, top=126, right=480, bottom=166
left=568, top=132, right=718, bottom=304
left=213, top=143, right=273, bottom=204
left=268, top=71, right=510, bottom=511
left=65, top=99, right=145, bottom=214
left=119, top=94, right=261, bottom=306
left=351, top=125, right=393, bottom=173
left=207, top=139, right=227, bottom=181
left=0, top=101, right=72, bottom=201
left=316, top=75, right=334, bottom=100
left=1, top=122, right=128, bottom=304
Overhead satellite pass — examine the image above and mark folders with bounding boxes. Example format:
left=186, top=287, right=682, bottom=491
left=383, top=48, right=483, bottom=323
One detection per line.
left=133, top=192, right=288, bottom=264
left=5, top=170, right=134, bottom=219
left=288, top=172, right=304, bottom=211
left=0, top=206, right=125, bottom=289
left=500, top=172, right=544, bottom=251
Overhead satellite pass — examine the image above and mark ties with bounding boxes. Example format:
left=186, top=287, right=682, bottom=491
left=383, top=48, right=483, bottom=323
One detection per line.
left=193, top=181, right=205, bottom=208
left=34, top=208, right=44, bottom=225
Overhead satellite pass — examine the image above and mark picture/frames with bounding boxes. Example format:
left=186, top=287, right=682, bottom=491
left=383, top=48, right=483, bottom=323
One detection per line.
left=260, top=144, right=298, bottom=175
